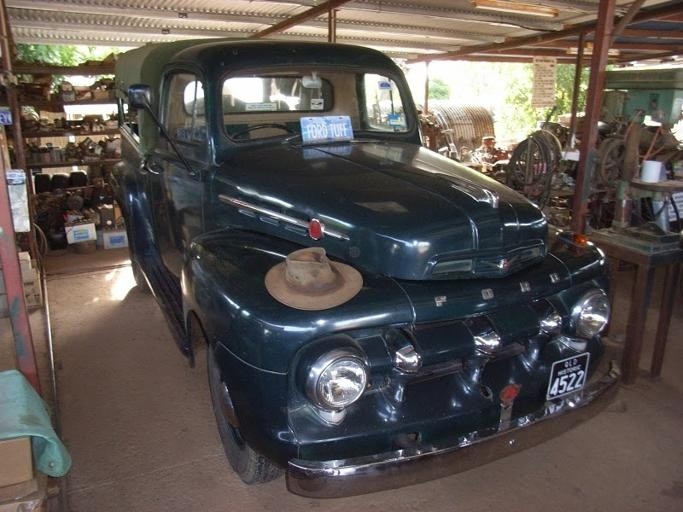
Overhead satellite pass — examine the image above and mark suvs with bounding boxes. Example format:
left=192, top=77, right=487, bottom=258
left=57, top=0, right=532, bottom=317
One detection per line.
left=108, top=35, right=623, bottom=500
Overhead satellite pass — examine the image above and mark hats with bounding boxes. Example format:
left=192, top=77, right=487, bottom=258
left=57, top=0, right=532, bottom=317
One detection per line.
left=264, top=246, right=364, bottom=311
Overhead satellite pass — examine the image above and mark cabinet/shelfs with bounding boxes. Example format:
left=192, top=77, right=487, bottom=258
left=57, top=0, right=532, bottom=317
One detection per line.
left=9, top=60, right=118, bottom=252
